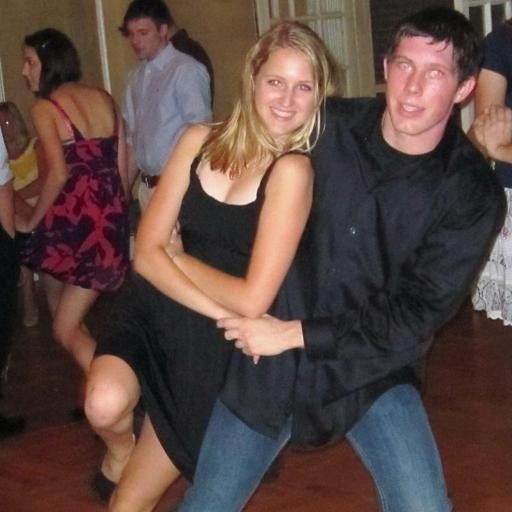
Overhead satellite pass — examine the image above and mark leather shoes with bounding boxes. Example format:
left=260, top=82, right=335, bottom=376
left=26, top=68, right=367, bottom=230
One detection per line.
left=23, top=317, right=33, bottom=328
left=141, top=173, right=160, bottom=188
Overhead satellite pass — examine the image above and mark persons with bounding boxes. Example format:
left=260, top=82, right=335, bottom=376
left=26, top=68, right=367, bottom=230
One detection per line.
left=117, top=1, right=215, bottom=257
left=175, top=6, right=512, bottom=512
left=13, top=27, right=132, bottom=427
left=1, top=101, right=61, bottom=328
left=166, top=8, right=215, bottom=107
left=1, top=131, right=26, bottom=440
left=83, top=20, right=338, bottom=511
left=464, top=15, right=512, bottom=333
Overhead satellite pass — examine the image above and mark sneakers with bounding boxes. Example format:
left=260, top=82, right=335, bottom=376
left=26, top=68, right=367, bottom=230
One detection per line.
left=0, top=415, right=25, bottom=442
left=94, top=469, right=116, bottom=502
